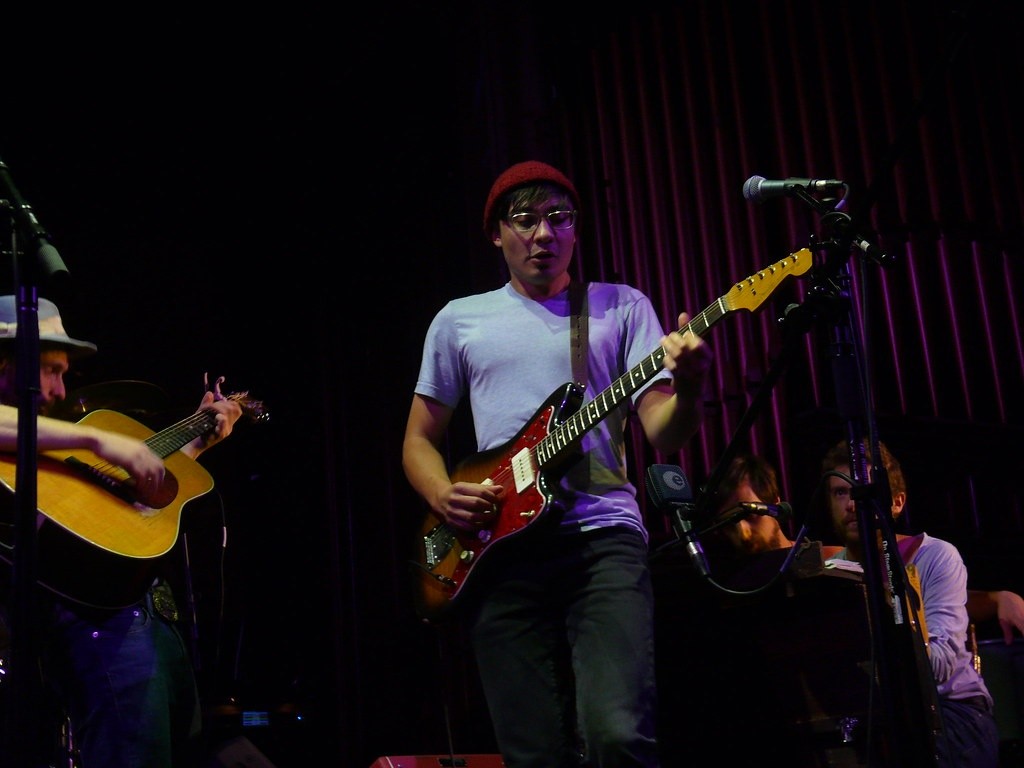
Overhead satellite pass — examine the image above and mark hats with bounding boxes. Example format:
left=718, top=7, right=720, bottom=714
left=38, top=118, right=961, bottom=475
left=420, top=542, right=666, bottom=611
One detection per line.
left=0, top=295, right=98, bottom=355
left=483, top=159, right=579, bottom=230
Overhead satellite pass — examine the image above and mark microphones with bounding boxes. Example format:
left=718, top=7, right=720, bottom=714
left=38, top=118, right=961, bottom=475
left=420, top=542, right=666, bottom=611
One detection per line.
left=743, top=176, right=844, bottom=202
left=645, top=464, right=712, bottom=577
left=741, top=501, right=784, bottom=518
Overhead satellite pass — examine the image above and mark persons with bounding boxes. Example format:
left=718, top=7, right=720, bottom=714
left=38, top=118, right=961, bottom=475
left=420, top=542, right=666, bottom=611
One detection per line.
left=0, top=296, right=242, bottom=768
left=690, top=436, right=1024, bottom=768
left=400, top=161, right=712, bottom=768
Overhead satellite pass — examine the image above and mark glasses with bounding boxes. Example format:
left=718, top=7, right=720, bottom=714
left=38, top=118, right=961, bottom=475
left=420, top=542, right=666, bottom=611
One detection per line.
left=499, top=210, right=575, bottom=231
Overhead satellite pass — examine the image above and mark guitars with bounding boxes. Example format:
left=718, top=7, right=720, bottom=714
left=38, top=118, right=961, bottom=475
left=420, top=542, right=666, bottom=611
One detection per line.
left=406, top=238, right=820, bottom=628
left=0, top=387, right=271, bottom=611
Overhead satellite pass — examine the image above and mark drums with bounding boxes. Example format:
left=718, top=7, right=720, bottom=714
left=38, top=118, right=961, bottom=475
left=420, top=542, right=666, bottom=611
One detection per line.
left=965, top=637, right=1024, bottom=756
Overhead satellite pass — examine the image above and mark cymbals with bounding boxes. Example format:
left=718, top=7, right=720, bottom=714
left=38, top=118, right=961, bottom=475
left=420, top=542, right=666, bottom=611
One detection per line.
left=72, top=377, right=171, bottom=425
